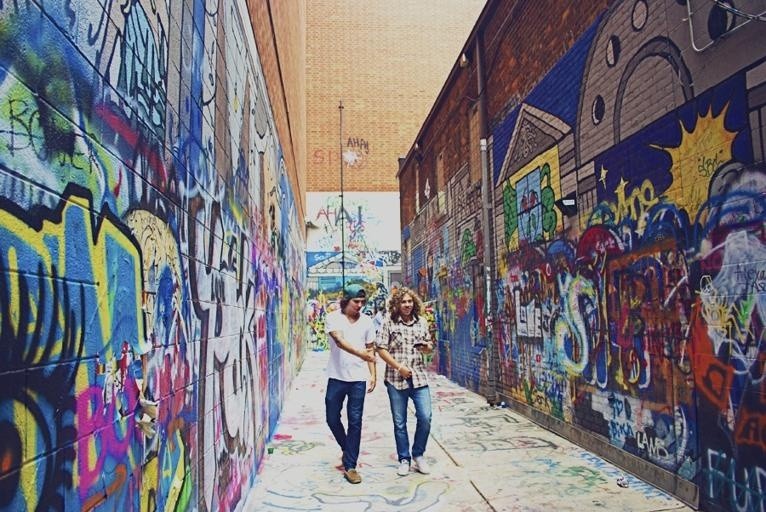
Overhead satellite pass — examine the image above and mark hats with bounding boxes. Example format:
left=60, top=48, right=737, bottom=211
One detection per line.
left=342, top=284, right=367, bottom=299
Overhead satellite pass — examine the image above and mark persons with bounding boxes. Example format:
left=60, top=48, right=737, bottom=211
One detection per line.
left=324, top=283, right=377, bottom=484
left=370, top=305, right=386, bottom=335
left=374, top=286, right=434, bottom=477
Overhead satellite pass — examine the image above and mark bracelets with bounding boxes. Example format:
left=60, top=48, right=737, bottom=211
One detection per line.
left=397, top=365, right=402, bottom=372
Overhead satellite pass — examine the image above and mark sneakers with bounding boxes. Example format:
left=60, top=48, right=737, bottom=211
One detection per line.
left=410, top=452, right=432, bottom=475
left=396, top=458, right=411, bottom=477
left=343, top=468, right=361, bottom=484
